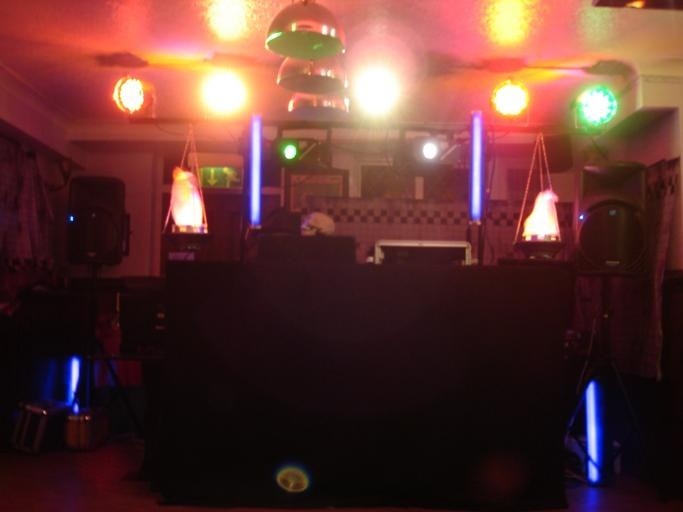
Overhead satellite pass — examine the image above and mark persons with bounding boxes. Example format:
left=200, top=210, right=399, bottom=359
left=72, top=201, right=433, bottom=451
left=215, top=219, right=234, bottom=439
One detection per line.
left=301, top=212, right=335, bottom=236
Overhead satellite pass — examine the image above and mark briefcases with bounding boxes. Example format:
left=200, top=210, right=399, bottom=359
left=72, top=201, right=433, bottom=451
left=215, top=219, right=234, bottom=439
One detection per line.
left=64, top=412, right=96, bottom=453
left=7, top=398, right=57, bottom=457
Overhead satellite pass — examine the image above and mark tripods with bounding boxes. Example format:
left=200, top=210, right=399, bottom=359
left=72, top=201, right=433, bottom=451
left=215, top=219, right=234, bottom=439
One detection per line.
left=560, top=275, right=667, bottom=502
left=47, top=265, right=145, bottom=453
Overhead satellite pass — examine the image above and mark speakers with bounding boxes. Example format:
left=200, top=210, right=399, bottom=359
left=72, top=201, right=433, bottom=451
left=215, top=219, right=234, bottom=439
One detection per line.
left=67, top=176, right=125, bottom=265
left=572, top=163, right=651, bottom=275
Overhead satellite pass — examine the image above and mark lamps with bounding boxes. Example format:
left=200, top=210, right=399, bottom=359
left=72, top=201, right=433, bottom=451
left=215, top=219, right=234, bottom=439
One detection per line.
left=268, top=1, right=350, bottom=115
